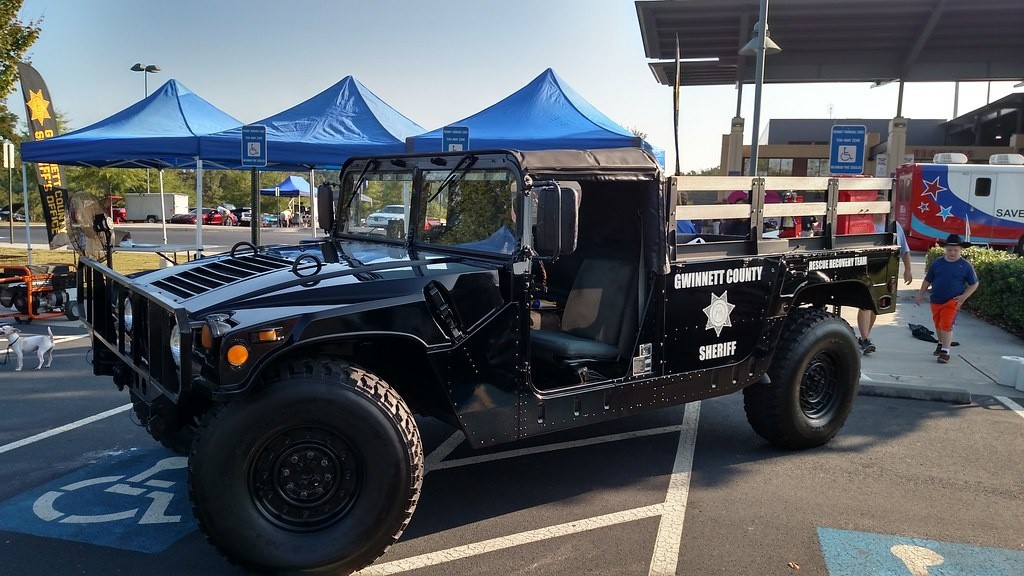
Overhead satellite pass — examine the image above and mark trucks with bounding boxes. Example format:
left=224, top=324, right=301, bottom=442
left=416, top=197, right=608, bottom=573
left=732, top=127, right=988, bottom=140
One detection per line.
left=104, top=192, right=188, bottom=224
left=893, top=152, right=1024, bottom=256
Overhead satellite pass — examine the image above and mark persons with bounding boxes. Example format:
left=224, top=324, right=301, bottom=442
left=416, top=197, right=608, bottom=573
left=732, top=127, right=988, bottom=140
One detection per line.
left=915, top=233, right=979, bottom=363
left=279, top=202, right=312, bottom=227
left=801, top=215, right=819, bottom=237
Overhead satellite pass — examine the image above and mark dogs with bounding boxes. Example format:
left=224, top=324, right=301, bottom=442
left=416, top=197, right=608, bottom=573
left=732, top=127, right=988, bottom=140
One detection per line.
left=0, top=325, right=55, bottom=371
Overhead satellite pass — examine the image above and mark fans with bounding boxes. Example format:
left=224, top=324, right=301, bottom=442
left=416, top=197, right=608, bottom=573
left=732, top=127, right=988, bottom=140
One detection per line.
left=66, top=191, right=115, bottom=269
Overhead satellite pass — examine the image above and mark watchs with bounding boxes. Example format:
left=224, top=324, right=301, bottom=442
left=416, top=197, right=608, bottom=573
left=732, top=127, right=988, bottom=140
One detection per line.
left=857, top=195, right=912, bottom=355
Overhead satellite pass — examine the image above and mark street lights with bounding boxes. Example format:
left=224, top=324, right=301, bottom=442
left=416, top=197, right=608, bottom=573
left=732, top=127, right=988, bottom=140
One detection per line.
left=130, top=64, right=162, bottom=193
left=738, top=0, right=783, bottom=204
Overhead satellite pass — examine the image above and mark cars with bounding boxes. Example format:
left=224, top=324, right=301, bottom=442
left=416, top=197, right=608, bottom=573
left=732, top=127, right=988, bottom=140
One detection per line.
left=171, top=207, right=238, bottom=227
left=366, top=205, right=412, bottom=229
left=0, top=203, right=32, bottom=222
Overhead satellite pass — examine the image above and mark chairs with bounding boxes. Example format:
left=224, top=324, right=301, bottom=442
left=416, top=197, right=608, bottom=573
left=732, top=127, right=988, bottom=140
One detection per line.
left=531, top=260, right=634, bottom=357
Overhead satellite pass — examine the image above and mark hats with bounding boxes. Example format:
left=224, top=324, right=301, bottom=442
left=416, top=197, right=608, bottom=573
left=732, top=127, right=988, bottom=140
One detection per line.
left=937, top=234, right=973, bottom=248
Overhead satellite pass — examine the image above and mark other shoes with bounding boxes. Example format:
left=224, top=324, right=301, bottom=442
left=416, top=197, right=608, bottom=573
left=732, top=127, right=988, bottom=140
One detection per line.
left=937, top=350, right=950, bottom=363
left=934, top=344, right=943, bottom=355
left=858, top=337, right=876, bottom=356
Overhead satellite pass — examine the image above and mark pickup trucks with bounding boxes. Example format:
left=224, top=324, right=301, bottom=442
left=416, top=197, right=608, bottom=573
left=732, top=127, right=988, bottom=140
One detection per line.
left=74, top=148, right=901, bottom=576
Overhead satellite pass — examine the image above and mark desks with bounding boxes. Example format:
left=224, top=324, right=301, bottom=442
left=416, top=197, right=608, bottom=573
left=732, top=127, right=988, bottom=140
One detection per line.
left=68, top=244, right=229, bottom=265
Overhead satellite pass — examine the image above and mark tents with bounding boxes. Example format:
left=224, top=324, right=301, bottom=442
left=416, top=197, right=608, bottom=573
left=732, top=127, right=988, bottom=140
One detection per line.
left=199, top=75, right=429, bottom=259
left=260, top=175, right=318, bottom=227
left=405, top=67, right=665, bottom=274
left=20, top=79, right=313, bottom=265
left=333, top=193, right=372, bottom=214
